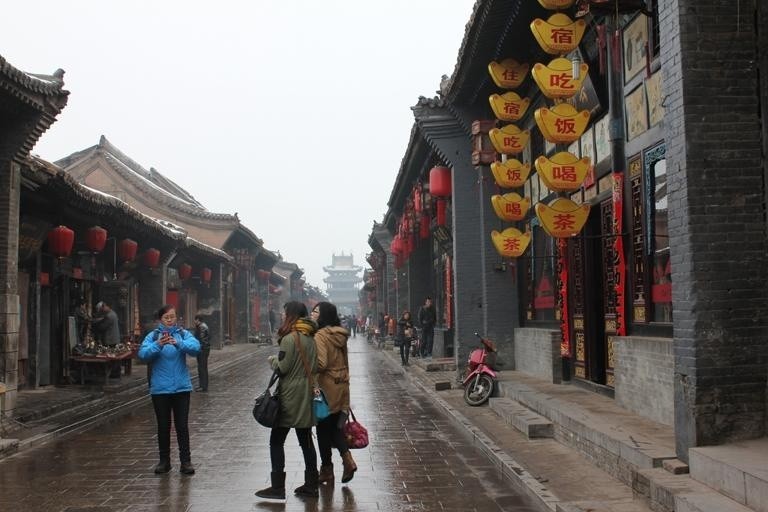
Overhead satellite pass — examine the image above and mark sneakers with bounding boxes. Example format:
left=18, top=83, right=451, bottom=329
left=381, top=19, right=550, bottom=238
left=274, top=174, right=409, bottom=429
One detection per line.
left=195, top=386, right=207, bottom=391
left=181, top=462, right=194, bottom=474
left=155, top=464, right=171, bottom=473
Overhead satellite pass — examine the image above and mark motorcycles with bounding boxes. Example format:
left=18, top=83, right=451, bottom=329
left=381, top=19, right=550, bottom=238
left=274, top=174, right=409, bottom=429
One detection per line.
left=462, top=331, right=500, bottom=404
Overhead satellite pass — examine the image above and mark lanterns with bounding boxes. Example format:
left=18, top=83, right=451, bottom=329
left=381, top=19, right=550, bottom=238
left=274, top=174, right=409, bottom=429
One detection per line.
left=85, top=222, right=107, bottom=255
left=48, top=223, right=75, bottom=262
left=143, top=247, right=160, bottom=272
left=180, top=262, right=195, bottom=279
left=389, top=162, right=452, bottom=268
left=120, top=237, right=138, bottom=264
left=367, top=289, right=377, bottom=309
left=256, top=268, right=272, bottom=282
left=200, top=268, right=212, bottom=285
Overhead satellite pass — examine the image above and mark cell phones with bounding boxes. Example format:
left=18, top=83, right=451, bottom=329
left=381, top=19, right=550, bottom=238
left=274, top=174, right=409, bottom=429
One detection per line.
left=162, top=330, right=170, bottom=344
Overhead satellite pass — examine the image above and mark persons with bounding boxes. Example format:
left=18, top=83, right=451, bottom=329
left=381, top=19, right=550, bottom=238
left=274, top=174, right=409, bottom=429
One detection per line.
left=397, top=310, right=414, bottom=366
left=138, top=304, right=201, bottom=474
left=338, top=310, right=394, bottom=344
left=255, top=301, right=320, bottom=502
left=194, top=315, right=210, bottom=392
left=137, top=310, right=161, bottom=391
left=270, top=307, right=275, bottom=334
left=418, top=297, right=437, bottom=358
left=309, top=302, right=357, bottom=484
left=90, top=301, right=121, bottom=382
left=72, top=299, right=104, bottom=344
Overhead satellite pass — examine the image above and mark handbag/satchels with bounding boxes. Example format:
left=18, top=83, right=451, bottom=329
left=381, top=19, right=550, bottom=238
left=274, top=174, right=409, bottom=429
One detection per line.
left=253, top=389, right=280, bottom=429
left=404, top=328, right=417, bottom=341
left=344, top=421, right=368, bottom=449
left=312, top=385, right=329, bottom=422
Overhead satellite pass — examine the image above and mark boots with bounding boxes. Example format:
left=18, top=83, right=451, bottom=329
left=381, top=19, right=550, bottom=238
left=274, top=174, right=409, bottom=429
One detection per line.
left=294, top=470, right=318, bottom=497
left=318, top=463, right=333, bottom=484
left=255, top=472, right=285, bottom=499
left=342, top=450, right=357, bottom=482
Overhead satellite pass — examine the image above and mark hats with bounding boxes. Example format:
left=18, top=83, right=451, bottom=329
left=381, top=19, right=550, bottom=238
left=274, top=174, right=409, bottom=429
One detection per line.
left=96, top=301, right=103, bottom=312
